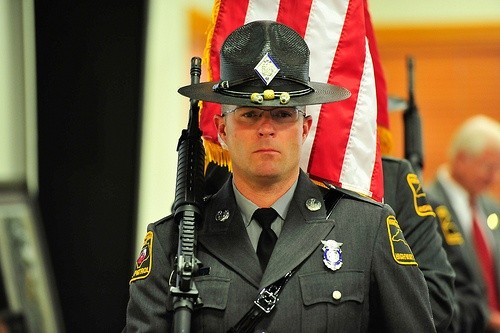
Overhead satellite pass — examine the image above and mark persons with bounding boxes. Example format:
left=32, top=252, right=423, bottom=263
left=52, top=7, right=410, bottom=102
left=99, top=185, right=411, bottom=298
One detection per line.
left=124, top=20, right=436, bottom=332
left=207, top=155, right=456, bottom=333
left=426, top=115, right=500, bottom=333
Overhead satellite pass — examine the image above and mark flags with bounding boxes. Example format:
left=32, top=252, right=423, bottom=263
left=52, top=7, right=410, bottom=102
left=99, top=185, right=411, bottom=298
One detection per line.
left=199, top=0, right=383, bottom=205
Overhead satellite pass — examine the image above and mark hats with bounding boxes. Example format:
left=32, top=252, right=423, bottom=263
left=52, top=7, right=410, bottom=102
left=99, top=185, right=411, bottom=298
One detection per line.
left=177, top=20, right=351, bottom=106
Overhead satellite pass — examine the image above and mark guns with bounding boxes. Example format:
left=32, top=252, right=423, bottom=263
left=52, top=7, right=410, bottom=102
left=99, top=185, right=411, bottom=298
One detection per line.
left=166, top=57, right=210, bottom=333
left=387, top=55, right=424, bottom=167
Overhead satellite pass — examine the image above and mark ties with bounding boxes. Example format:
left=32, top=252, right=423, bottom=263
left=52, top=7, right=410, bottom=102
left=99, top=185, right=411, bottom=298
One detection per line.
left=253, top=207, right=279, bottom=273
left=470, top=205, right=499, bottom=316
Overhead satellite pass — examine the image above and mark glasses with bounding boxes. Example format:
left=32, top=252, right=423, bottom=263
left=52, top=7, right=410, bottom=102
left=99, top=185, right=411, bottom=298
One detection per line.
left=222, top=106, right=306, bottom=123
